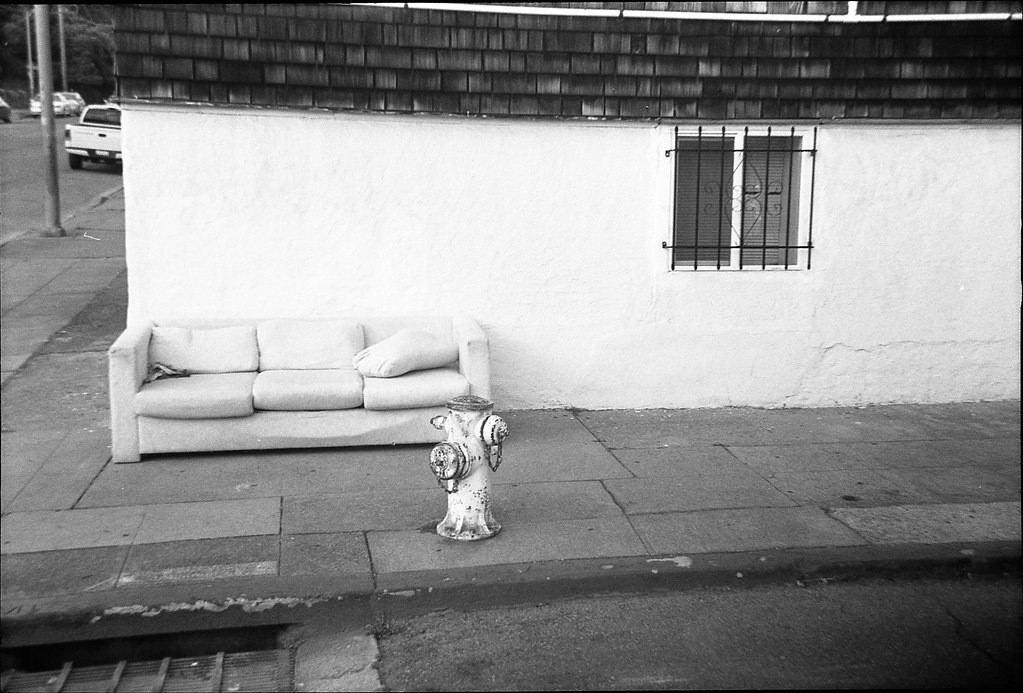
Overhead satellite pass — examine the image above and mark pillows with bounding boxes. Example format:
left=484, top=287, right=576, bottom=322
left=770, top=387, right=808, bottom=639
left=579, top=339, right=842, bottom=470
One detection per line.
left=353, top=330, right=458, bottom=378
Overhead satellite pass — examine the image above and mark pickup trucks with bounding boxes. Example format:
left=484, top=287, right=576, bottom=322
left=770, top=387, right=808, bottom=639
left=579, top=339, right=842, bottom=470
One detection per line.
left=65, top=104, right=122, bottom=170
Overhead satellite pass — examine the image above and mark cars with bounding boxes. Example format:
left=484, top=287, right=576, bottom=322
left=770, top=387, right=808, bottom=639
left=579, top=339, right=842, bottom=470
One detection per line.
left=30, top=91, right=86, bottom=117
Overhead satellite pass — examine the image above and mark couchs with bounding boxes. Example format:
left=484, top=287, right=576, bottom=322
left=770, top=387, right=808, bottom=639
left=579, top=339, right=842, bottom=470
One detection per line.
left=108, top=309, right=492, bottom=464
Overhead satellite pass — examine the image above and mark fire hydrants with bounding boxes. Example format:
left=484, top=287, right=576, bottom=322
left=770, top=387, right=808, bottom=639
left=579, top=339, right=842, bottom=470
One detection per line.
left=428, top=394, right=510, bottom=542
